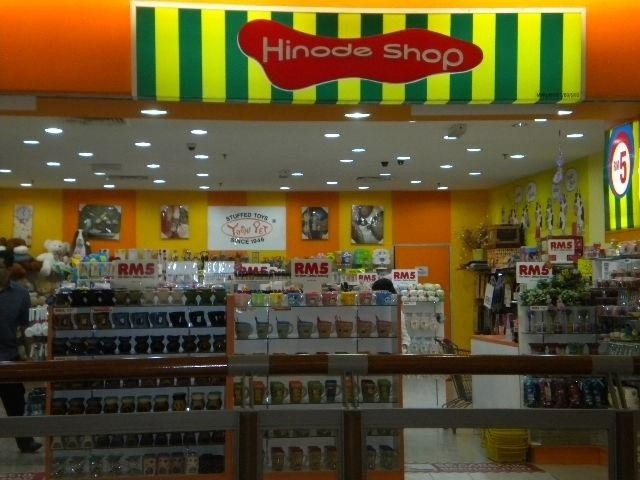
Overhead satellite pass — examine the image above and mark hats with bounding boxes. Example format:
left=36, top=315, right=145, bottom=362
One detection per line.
left=371, top=279, right=397, bottom=294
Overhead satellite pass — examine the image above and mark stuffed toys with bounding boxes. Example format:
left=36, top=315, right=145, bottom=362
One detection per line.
left=72, top=228, right=91, bottom=255
left=0, top=236, right=72, bottom=282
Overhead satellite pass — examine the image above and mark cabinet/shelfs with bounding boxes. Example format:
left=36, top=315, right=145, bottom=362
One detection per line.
left=518, top=255, right=640, bottom=411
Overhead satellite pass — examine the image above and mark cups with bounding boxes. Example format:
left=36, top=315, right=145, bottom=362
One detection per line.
left=48, top=430, right=225, bottom=451
left=271, top=444, right=396, bottom=474
left=231, top=378, right=390, bottom=404
left=232, top=317, right=394, bottom=339
left=198, top=452, right=226, bottom=475
left=233, top=291, right=393, bottom=306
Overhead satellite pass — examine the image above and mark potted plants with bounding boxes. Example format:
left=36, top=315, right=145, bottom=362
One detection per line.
left=453, top=222, right=488, bottom=261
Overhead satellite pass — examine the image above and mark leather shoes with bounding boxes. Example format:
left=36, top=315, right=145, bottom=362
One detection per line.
left=21, top=442, right=42, bottom=454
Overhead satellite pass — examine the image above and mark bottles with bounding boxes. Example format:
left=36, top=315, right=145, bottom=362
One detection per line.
left=142, top=451, right=201, bottom=476
left=73, top=228, right=92, bottom=257
left=49, top=391, right=224, bottom=416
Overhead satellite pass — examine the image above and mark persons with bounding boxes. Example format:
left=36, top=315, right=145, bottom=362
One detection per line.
left=372, top=278, right=412, bottom=354
left=1, top=250, right=46, bottom=454
